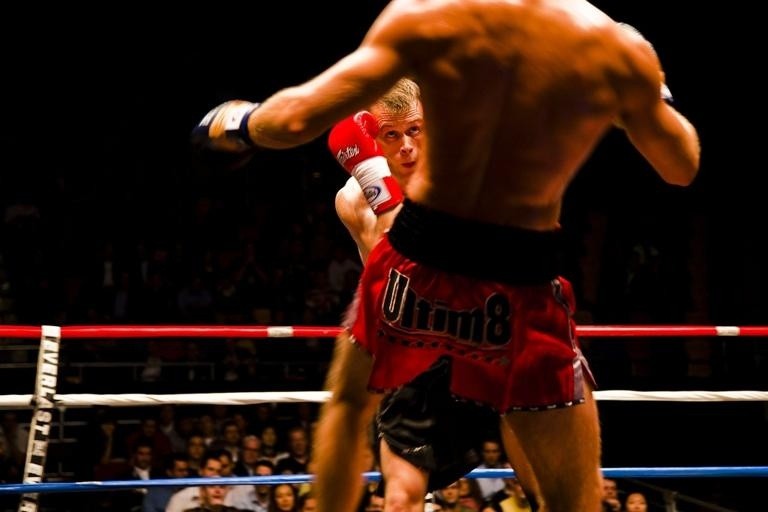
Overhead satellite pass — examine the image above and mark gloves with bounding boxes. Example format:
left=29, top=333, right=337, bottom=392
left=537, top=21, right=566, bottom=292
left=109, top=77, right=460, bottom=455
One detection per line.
left=197, top=98, right=261, bottom=155
left=328, top=111, right=405, bottom=216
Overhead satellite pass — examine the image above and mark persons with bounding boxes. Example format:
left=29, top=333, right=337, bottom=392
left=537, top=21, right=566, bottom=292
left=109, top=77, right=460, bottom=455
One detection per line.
left=191, top=0, right=702, bottom=512
left=0, top=242, right=768, bottom=512
left=326, top=75, right=547, bottom=512
